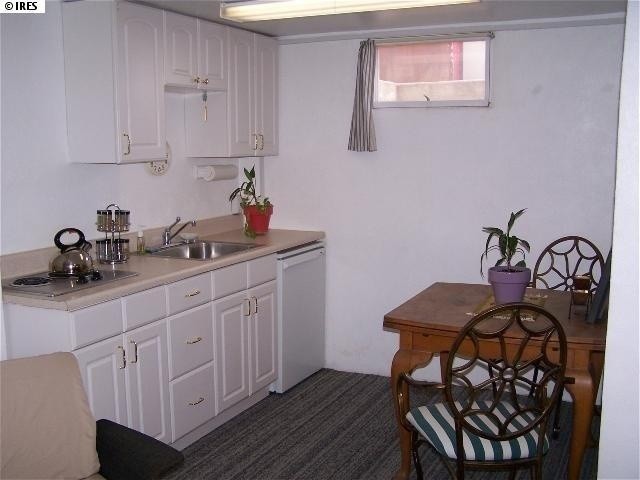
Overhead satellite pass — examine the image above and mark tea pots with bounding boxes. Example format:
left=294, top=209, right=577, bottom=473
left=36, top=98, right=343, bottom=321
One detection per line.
left=48, top=228, right=94, bottom=275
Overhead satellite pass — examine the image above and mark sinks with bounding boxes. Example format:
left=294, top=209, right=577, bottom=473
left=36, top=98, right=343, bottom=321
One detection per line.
left=151, top=238, right=267, bottom=263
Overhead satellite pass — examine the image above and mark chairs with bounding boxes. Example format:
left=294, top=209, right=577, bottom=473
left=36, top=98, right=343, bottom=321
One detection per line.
left=488, top=235, right=604, bottom=440
left=1, top=351, right=184, bottom=478
left=401, top=301, right=576, bottom=480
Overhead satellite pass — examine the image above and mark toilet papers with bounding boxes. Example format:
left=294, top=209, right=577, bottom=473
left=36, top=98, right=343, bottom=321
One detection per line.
left=205, top=165, right=238, bottom=183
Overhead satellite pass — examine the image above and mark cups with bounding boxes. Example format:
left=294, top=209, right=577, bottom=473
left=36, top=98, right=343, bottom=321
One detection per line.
left=97, top=210, right=130, bottom=260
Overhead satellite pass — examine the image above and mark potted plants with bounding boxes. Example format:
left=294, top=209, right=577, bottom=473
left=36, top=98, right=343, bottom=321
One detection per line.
left=229, top=165, right=274, bottom=241
left=480, top=206, right=531, bottom=309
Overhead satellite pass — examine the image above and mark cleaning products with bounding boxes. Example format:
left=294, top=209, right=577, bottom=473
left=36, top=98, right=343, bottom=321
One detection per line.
left=137, top=226, right=146, bottom=253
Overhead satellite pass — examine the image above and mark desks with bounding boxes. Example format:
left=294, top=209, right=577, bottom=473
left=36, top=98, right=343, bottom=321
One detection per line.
left=382, top=282, right=607, bottom=479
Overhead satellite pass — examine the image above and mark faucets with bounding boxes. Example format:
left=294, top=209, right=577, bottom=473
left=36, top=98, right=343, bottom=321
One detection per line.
left=164, top=218, right=196, bottom=245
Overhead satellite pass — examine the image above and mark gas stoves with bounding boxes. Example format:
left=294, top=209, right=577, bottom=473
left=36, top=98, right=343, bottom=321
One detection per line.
left=1, top=269, right=139, bottom=298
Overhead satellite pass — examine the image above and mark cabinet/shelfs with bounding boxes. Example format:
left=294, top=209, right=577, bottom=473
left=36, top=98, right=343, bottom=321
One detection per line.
left=185, top=25, right=280, bottom=158
left=211, top=252, right=279, bottom=417
left=164, top=9, right=228, bottom=91
left=2, top=285, right=172, bottom=447
left=62, top=0, right=168, bottom=166
left=164, top=272, right=216, bottom=444
